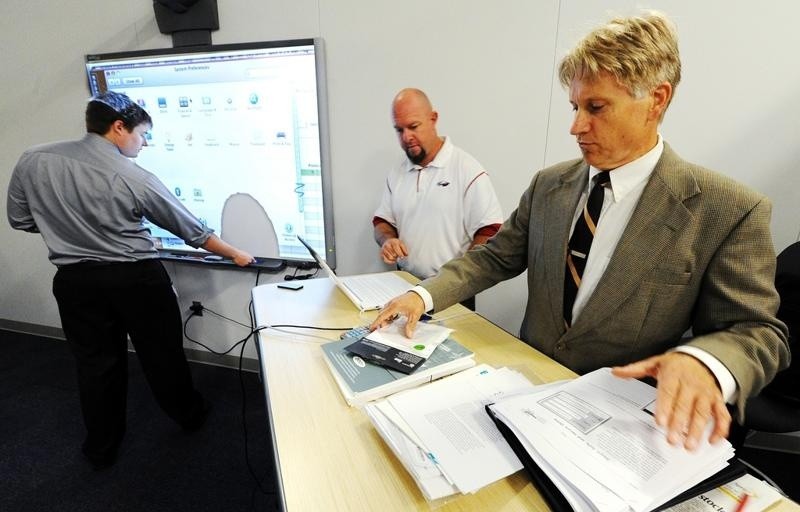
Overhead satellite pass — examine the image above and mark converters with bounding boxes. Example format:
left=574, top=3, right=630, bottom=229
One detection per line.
left=189, top=304, right=204, bottom=312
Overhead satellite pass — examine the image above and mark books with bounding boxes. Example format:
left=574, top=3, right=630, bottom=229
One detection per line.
left=320, top=322, right=783, bottom=510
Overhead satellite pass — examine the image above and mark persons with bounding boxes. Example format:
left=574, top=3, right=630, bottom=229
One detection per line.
left=368, top=10, right=793, bottom=451
left=8, top=92, right=256, bottom=470
left=371, top=87, right=503, bottom=313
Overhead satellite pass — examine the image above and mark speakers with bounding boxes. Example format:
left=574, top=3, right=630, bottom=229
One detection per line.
left=152, top=1, right=224, bottom=50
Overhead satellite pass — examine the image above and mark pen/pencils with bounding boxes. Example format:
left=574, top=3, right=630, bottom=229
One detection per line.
left=736, top=494, right=749, bottom=512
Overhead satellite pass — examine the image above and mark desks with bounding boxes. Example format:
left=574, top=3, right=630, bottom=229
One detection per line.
left=251, top=269, right=800, bottom=511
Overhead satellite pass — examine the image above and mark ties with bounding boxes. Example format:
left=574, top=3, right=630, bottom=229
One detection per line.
left=564, top=170, right=609, bottom=327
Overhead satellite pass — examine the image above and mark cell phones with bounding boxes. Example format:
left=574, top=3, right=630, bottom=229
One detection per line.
left=278, top=282, right=304, bottom=291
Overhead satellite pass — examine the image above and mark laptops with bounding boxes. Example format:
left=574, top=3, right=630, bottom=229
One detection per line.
left=296, top=234, right=417, bottom=315
